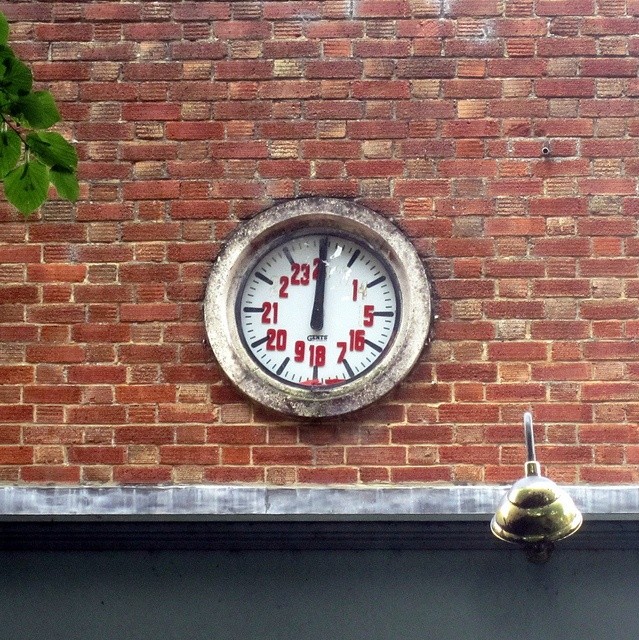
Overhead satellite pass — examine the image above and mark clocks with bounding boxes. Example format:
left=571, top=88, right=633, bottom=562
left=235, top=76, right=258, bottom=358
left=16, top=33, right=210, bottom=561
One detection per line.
left=201, top=197, right=432, bottom=427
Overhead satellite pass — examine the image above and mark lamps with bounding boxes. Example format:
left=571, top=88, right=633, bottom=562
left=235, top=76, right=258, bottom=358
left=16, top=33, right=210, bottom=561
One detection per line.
left=489, top=413, right=584, bottom=550
